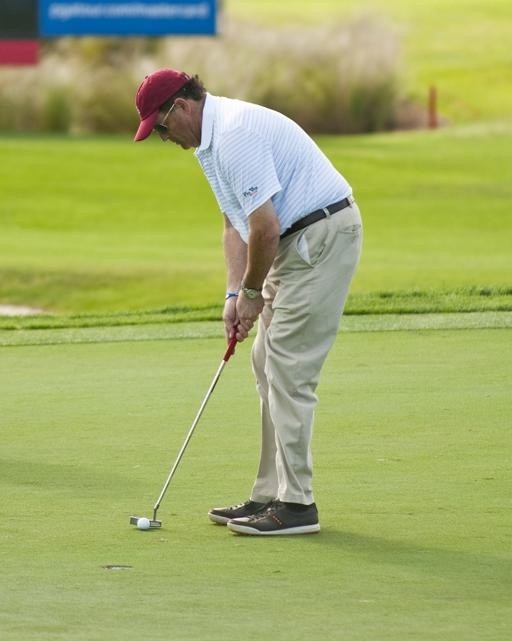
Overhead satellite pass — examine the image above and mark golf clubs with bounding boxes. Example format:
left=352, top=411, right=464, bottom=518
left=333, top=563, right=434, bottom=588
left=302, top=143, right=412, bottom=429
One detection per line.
left=129, top=325, right=241, bottom=529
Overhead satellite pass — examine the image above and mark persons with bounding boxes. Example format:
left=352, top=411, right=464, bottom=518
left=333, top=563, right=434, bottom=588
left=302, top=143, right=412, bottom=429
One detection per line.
left=131, top=67, right=366, bottom=537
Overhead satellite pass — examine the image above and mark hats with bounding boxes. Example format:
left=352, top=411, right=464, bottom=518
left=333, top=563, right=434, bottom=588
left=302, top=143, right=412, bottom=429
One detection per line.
left=134, top=68, right=192, bottom=143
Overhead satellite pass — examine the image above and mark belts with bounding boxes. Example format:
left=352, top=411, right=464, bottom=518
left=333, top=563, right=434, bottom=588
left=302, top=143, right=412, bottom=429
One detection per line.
left=278, top=199, right=349, bottom=240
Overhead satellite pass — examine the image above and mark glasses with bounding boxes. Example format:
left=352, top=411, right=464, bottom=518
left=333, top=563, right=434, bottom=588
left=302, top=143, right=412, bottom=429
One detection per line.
left=152, top=102, right=176, bottom=135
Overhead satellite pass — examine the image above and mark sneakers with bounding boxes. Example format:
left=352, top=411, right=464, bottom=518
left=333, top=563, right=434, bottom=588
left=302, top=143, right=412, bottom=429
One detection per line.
left=209, top=499, right=273, bottom=525
left=227, top=498, right=321, bottom=535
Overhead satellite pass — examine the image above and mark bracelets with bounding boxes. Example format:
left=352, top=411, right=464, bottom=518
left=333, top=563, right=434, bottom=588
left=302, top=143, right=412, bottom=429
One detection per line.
left=224, top=289, right=240, bottom=299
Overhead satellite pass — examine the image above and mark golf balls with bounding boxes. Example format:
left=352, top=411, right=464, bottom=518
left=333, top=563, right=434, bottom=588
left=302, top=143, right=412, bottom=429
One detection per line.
left=137, top=518, right=150, bottom=530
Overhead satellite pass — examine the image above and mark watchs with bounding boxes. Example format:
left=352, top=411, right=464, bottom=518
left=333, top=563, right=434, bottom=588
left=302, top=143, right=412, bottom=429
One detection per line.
left=241, top=287, right=262, bottom=299
